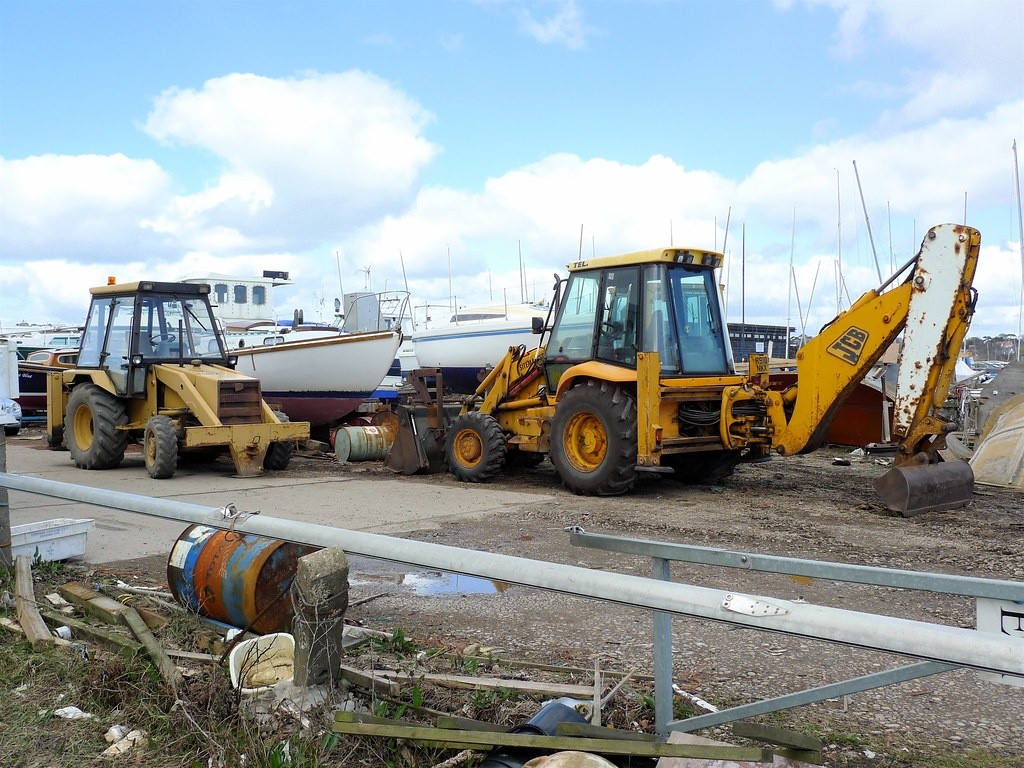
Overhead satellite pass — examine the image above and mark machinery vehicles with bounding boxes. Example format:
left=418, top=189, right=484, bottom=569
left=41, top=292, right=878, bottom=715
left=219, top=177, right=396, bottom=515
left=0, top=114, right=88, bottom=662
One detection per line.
left=382, top=223, right=981, bottom=517
left=46, top=276, right=311, bottom=479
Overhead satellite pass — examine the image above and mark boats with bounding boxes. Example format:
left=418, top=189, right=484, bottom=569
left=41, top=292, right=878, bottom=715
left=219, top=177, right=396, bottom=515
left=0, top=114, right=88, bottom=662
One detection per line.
left=0, top=322, right=85, bottom=352
left=971, top=359, right=1010, bottom=384
left=664, top=320, right=945, bottom=453
left=12, top=278, right=399, bottom=428
left=409, top=306, right=609, bottom=399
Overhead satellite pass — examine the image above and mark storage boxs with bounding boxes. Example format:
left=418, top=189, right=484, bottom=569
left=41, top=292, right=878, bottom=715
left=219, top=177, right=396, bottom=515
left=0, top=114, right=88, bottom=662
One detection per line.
left=11, top=517, right=95, bottom=565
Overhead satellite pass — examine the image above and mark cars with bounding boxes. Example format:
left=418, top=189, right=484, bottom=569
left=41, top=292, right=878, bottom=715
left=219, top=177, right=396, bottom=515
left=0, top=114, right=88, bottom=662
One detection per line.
left=0, top=395, right=22, bottom=436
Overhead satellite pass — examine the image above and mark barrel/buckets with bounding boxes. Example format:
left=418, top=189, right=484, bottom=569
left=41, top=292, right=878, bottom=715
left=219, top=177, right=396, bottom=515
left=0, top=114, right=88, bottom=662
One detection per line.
left=167, top=524, right=323, bottom=635
left=334, top=425, right=394, bottom=461
left=478, top=701, right=588, bottom=768
left=230, top=632, right=297, bottom=695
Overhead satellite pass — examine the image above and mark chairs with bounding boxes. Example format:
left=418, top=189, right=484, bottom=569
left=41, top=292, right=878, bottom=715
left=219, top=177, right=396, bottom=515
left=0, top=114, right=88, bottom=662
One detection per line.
left=646, top=309, right=664, bottom=346
left=139, top=330, right=154, bottom=356
